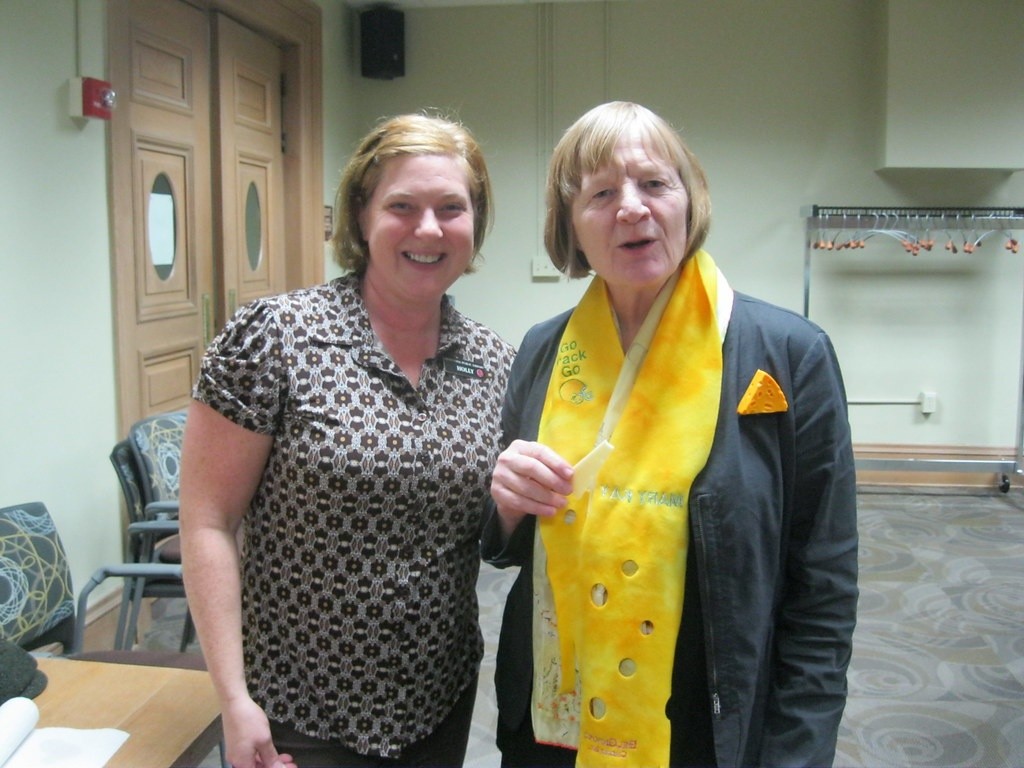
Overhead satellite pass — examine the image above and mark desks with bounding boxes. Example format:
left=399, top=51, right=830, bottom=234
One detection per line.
left=0, top=657, right=224, bottom=768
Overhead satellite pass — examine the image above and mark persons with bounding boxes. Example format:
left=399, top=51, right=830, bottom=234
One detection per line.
left=479, top=101, right=858, bottom=768
left=177, top=114, right=519, bottom=768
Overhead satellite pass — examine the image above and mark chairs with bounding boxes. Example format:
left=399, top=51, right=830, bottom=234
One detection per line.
left=0, top=409, right=210, bottom=668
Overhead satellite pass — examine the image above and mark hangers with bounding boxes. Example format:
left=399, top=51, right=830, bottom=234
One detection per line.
left=815, top=209, right=1024, bottom=256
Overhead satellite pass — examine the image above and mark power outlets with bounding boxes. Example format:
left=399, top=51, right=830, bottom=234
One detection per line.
left=531, top=261, right=559, bottom=280
